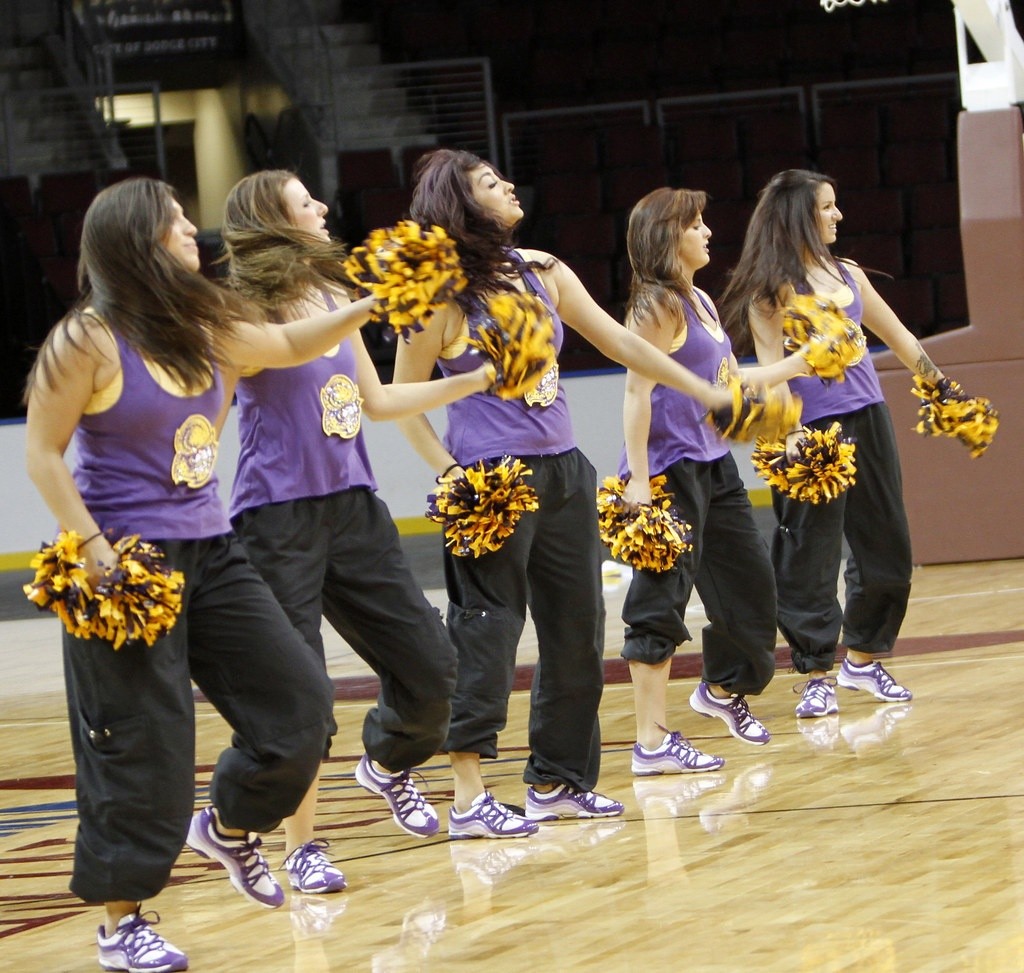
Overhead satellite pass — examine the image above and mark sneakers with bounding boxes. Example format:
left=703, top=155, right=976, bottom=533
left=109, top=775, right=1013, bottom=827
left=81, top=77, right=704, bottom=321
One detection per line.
left=631, top=732, right=725, bottom=775
left=836, top=657, right=912, bottom=702
left=97, top=904, right=188, bottom=973
left=840, top=702, right=912, bottom=750
left=689, top=681, right=770, bottom=745
left=795, top=680, right=838, bottom=717
left=449, top=791, right=539, bottom=838
left=633, top=770, right=727, bottom=817
left=450, top=842, right=540, bottom=885
left=355, top=752, right=439, bottom=839
left=289, top=894, right=349, bottom=935
left=525, top=784, right=624, bottom=821
left=186, top=805, right=284, bottom=908
left=371, top=900, right=446, bottom=970
left=796, top=716, right=840, bottom=750
left=285, top=838, right=347, bottom=894
left=540, top=819, right=626, bottom=854
left=699, top=762, right=773, bottom=836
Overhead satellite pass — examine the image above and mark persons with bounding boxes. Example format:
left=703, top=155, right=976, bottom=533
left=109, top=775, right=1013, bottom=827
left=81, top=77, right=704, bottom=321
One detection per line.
left=385, top=146, right=810, bottom=840
left=711, top=169, right=1000, bottom=716
left=208, top=169, right=550, bottom=898
left=20, top=179, right=468, bottom=973
left=595, top=186, right=861, bottom=772
